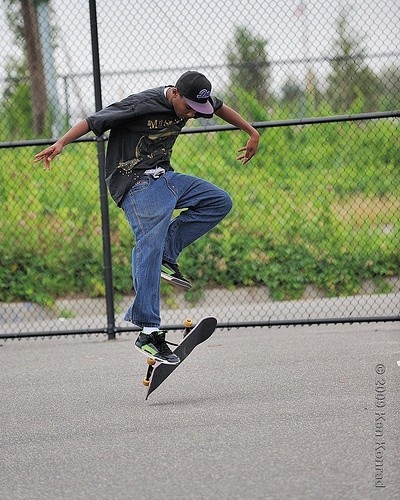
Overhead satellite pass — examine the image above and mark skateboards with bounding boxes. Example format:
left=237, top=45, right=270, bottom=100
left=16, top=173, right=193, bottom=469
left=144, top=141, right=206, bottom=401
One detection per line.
left=143, top=317, right=217, bottom=400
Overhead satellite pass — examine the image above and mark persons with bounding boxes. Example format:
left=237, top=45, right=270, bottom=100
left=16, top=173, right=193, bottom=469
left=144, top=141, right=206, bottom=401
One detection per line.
left=34, top=70, right=260, bottom=366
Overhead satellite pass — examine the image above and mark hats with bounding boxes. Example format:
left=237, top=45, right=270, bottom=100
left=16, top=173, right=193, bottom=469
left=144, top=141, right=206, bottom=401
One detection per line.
left=176, top=71, right=214, bottom=115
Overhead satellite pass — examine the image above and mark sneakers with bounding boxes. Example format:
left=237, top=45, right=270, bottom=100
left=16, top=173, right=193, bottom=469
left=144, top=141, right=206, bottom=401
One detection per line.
left=161, top=259, right=192, bottom=289
left=134, top=330, right=181, bottom=366
